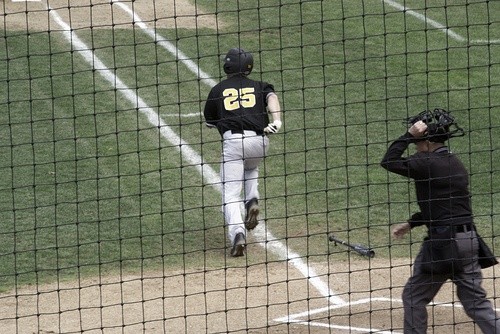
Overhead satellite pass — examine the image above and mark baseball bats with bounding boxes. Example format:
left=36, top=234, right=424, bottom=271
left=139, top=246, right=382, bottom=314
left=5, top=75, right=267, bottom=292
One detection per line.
left=328, top=233, right=375, bottom=258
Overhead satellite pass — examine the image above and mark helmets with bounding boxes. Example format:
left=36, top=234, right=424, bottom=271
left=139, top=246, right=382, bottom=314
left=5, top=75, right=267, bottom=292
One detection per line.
left=223, top=48, right=254, bottom=75
left=410, top=107, right=465, bottom=143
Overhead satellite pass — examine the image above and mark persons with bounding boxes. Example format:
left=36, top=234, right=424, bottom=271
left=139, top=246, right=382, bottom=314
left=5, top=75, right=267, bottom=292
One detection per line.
left=379, top=107, right=500, bottom=334
left=204, top=49, right=282, bottom=257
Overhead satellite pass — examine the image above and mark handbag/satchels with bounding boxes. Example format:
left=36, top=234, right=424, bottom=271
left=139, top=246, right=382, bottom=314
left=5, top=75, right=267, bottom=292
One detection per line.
left=422, top=226, right=464, bottom=273
left=477, top=235, right=499, bottom=268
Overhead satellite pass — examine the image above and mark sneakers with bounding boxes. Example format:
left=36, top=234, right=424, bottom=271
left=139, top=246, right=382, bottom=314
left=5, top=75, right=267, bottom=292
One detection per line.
left=231, top=233, right=246, bottom=256
left=245, top=198, right=259, bottom=231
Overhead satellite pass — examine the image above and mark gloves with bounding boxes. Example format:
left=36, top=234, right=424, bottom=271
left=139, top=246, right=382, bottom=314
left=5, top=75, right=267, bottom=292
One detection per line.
left=264, top=120, right=282, bottom=133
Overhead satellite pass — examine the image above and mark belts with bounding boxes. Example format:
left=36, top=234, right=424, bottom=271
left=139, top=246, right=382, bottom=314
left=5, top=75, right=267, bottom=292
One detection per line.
left=231, top=129, right=263, bottom=135
left=426, top=224, right=472, bottom=236
